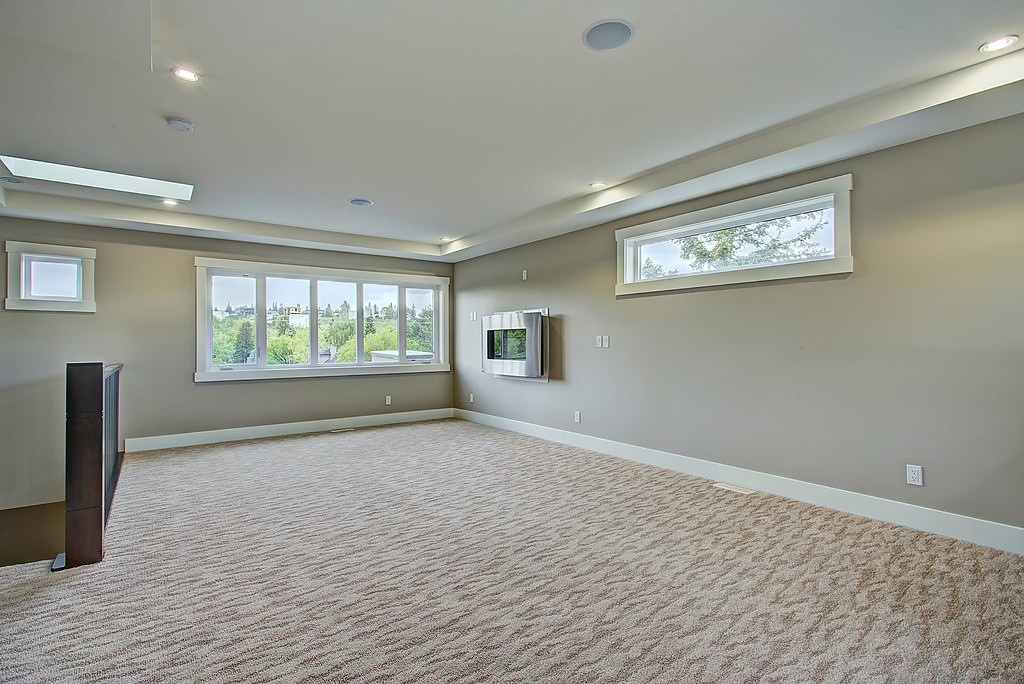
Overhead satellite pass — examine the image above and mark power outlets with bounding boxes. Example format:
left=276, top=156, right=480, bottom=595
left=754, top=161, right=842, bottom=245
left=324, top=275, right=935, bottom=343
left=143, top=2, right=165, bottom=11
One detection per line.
left=469, top=394, right=473, bottom=403
left=386, top=395, right=391, bottom=405
left=906, top=464, right=923, bottom=486
left=522, top=269, right=527, bottom=280
left=575, top=410, right=581, bottom=423
